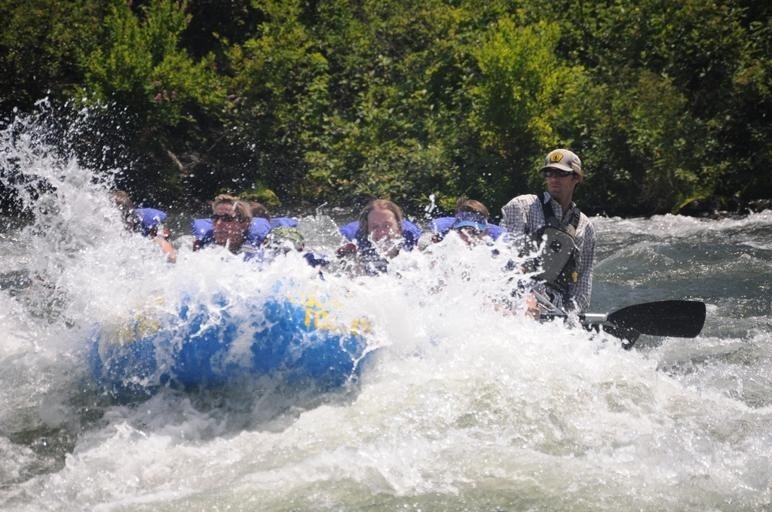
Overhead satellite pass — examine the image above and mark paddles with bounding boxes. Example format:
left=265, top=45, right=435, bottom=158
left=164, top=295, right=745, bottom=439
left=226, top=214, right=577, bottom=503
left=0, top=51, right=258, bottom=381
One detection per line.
left=531, top=299, right=706, bottom=338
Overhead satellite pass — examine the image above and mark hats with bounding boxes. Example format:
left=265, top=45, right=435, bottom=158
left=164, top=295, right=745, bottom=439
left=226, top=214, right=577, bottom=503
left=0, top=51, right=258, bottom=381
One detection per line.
left=540, top=148, right=582, bottom=176
left=269, top=226, right=303, bottom=241
left=453, top=213, right=485, bottom=231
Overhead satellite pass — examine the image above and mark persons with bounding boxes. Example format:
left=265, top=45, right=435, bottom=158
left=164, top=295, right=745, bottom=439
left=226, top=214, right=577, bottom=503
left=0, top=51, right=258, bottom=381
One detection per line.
left=332, top=200, right=422, bottom=277
left=105, top=186, right=166, bottom=239
left=147, top=193, right=269, bottom=267
left=454, top=199, right=490, bottom=225
left=492, top=147, right=596, bottom=323
left=241, top=199, right=272, bottom=223
left=431, top=219, right=499, bottom=254
left=258, top=227, right=328, bottom=280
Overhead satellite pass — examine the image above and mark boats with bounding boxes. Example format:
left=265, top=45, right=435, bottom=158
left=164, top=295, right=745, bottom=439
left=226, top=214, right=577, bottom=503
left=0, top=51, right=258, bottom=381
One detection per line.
left=84, top=273, right=390, bottom=404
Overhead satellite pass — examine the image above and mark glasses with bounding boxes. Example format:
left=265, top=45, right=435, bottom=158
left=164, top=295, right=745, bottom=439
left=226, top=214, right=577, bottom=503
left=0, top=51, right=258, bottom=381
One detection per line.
left=210, top=212, right=235, bottom=223
left=545, top=167, right=572, bottom=177
